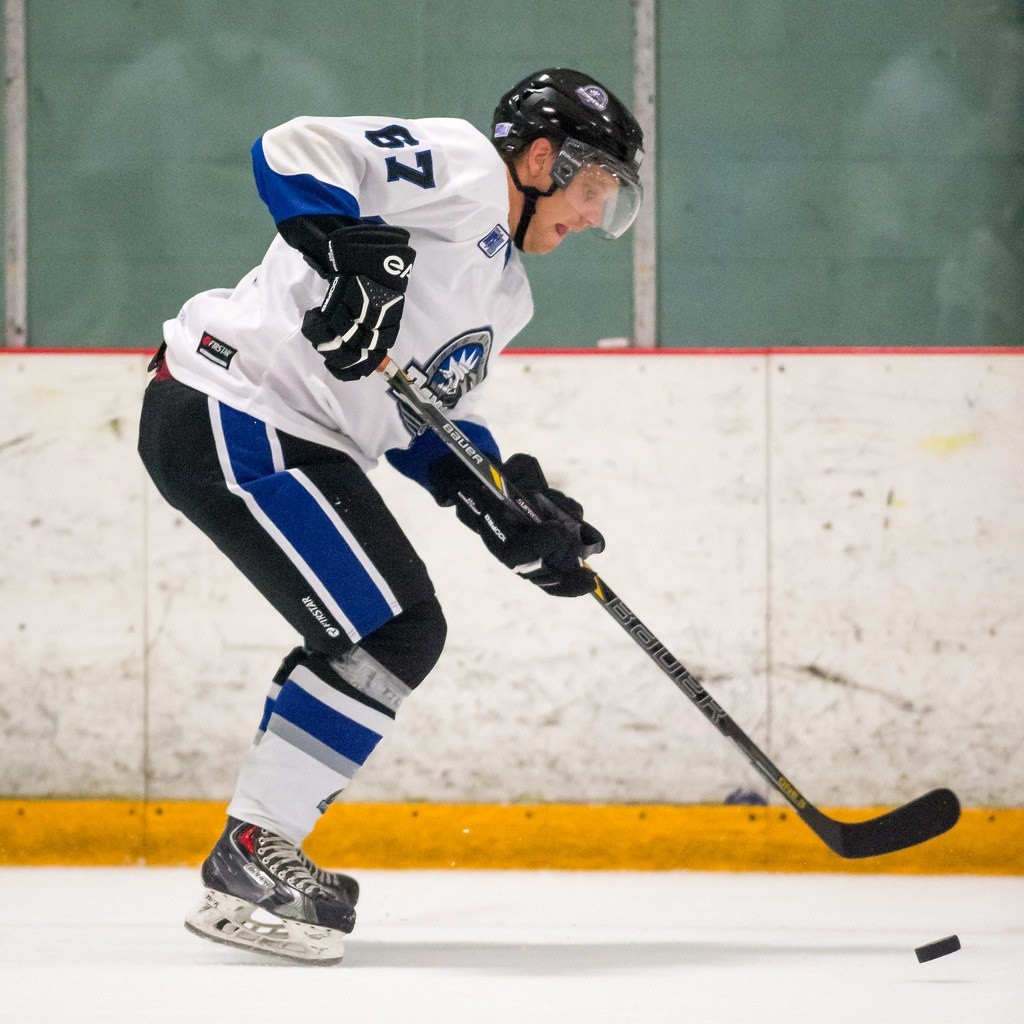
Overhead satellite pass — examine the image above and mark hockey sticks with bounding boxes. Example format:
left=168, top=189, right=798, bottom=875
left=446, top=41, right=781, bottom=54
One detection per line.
left=376, top=352, right=962, bottom=863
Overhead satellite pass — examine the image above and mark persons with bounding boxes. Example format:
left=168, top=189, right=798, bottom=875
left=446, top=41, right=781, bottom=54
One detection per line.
left=137, top=68, right=644, bottom=935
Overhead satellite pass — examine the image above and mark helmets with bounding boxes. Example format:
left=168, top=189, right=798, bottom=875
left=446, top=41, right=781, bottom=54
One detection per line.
left=492, top=68, right=644, bottom=186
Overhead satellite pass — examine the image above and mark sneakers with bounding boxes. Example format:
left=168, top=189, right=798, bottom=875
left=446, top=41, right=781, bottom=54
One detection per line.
left=185, top=817, right=354, bottom=963
left=297, top=849, right=359, bottom=907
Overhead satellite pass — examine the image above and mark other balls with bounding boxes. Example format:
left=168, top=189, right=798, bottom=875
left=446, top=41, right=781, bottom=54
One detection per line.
left=914, top=934, right=961, bottom=964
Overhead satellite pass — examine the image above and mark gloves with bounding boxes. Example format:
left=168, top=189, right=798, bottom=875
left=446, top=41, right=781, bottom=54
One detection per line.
left=455, top=453, right=605, bottom=598
left=303, top=224, right=418, bottom=380
